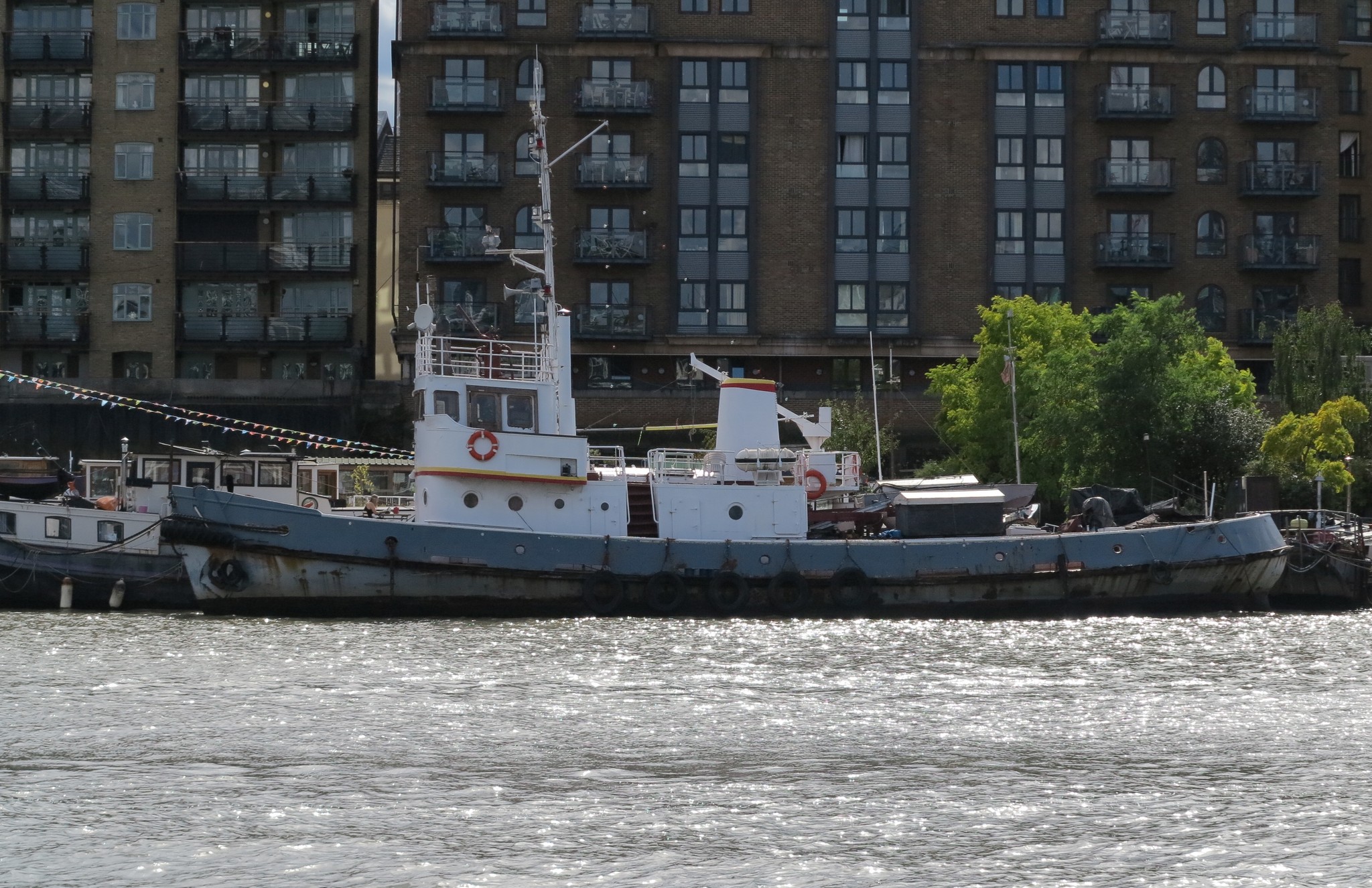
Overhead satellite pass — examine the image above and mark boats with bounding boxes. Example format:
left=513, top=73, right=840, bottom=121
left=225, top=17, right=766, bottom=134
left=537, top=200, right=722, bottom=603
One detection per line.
left=1234, top=509, right=1372, bottom=609
left=0, top=437, right=416, bottom=612
left=157, top=37, right=1294, bottom=617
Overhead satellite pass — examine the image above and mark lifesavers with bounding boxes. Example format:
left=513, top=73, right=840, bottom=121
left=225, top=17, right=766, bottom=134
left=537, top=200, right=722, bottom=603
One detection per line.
left=467, top=431, right=498, bottom=461
left=800, top=470, right=827, bottom=499
left=207, top=559, right=248, bottom=592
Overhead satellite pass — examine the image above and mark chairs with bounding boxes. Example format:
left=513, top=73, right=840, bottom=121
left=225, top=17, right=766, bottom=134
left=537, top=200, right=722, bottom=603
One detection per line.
left=362, top=510, right=369, bottom=518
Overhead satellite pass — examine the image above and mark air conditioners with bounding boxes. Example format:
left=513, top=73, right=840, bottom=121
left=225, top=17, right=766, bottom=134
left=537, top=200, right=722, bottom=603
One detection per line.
left=676, top=358, right=704, bottom=381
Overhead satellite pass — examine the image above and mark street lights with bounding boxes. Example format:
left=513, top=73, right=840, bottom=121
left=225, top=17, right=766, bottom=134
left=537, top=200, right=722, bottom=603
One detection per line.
left=1142, top=433, right=1154, bottom=504
left=1315, top=472, right=1325, bottom=528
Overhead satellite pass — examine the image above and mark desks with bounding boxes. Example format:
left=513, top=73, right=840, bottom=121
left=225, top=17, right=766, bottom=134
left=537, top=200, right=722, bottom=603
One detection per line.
left=376, top=513, right=411, bottom=522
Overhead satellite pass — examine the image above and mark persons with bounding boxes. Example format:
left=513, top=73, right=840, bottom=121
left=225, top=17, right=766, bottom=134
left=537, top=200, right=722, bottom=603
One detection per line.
left=363, top=494, right=391, bottom=518
